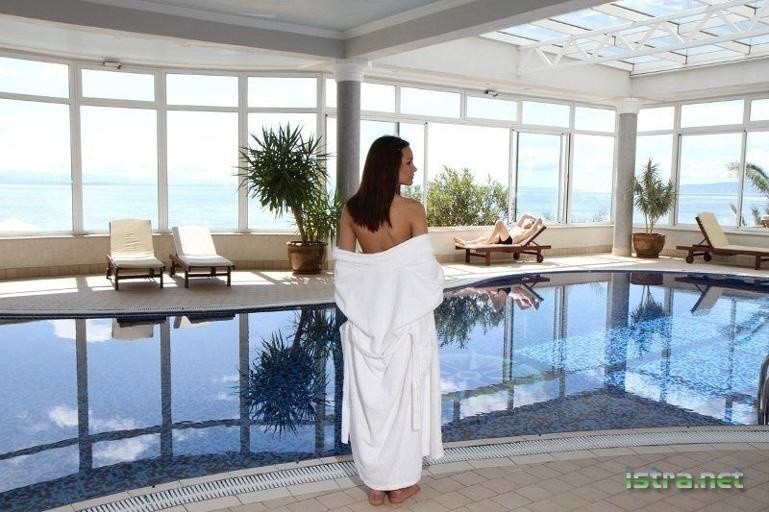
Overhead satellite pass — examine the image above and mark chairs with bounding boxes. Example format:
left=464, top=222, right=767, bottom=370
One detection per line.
left=672, top=274, right=769, bottom=316
left=455, top=223, right=554, bottom=264
left=452, top=276, right=551, bottom=309
left=106, top=215, right=236, bottom=289
left=675, top=210, right=769, bottom=269
left=110, top=310, right=236, bottom=341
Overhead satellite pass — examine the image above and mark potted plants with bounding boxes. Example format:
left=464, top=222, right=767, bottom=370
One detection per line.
left=240, top=127, right=346, bottom=275
left=623, top=157, right=680, bottom=259
left=622, top=270, right=672, bottom=361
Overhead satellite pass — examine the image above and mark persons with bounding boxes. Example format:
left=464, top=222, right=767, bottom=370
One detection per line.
left=452, top=212, right=545, bottom=245
left=330, top=134, right=446, bottom=506
left=447, top=285, right=540, bottom=314
